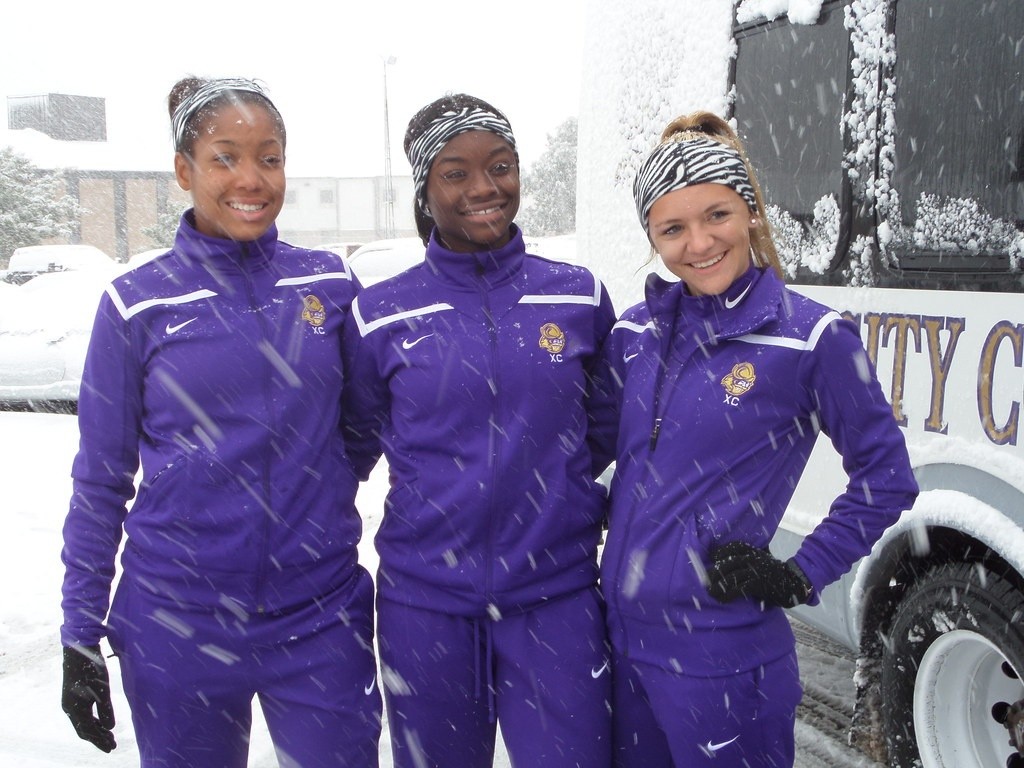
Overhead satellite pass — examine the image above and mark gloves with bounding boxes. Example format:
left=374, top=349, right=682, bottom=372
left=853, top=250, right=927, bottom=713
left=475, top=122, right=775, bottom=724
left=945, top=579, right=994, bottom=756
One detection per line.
left=60, top=643, right=117, bottom=753
left=707, top=542, right=806, bottom=609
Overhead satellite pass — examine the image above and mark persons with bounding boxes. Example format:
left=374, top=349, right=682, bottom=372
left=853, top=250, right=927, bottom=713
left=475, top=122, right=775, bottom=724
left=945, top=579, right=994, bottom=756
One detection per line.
left=61, top=75, right=383, bottom=768
left=586, top=112, right=923, bottom=768
left=335, top=95, right=619, bottom=768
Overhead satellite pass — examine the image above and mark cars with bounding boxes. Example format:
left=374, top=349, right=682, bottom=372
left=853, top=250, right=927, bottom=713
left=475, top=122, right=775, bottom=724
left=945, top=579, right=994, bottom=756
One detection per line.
left=0, top=235, right=544, bottom=412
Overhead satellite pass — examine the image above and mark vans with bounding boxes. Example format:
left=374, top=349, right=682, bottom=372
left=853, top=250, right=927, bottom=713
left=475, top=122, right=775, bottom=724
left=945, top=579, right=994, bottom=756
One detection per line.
left=574, top=2, right=1024, bottom=768
